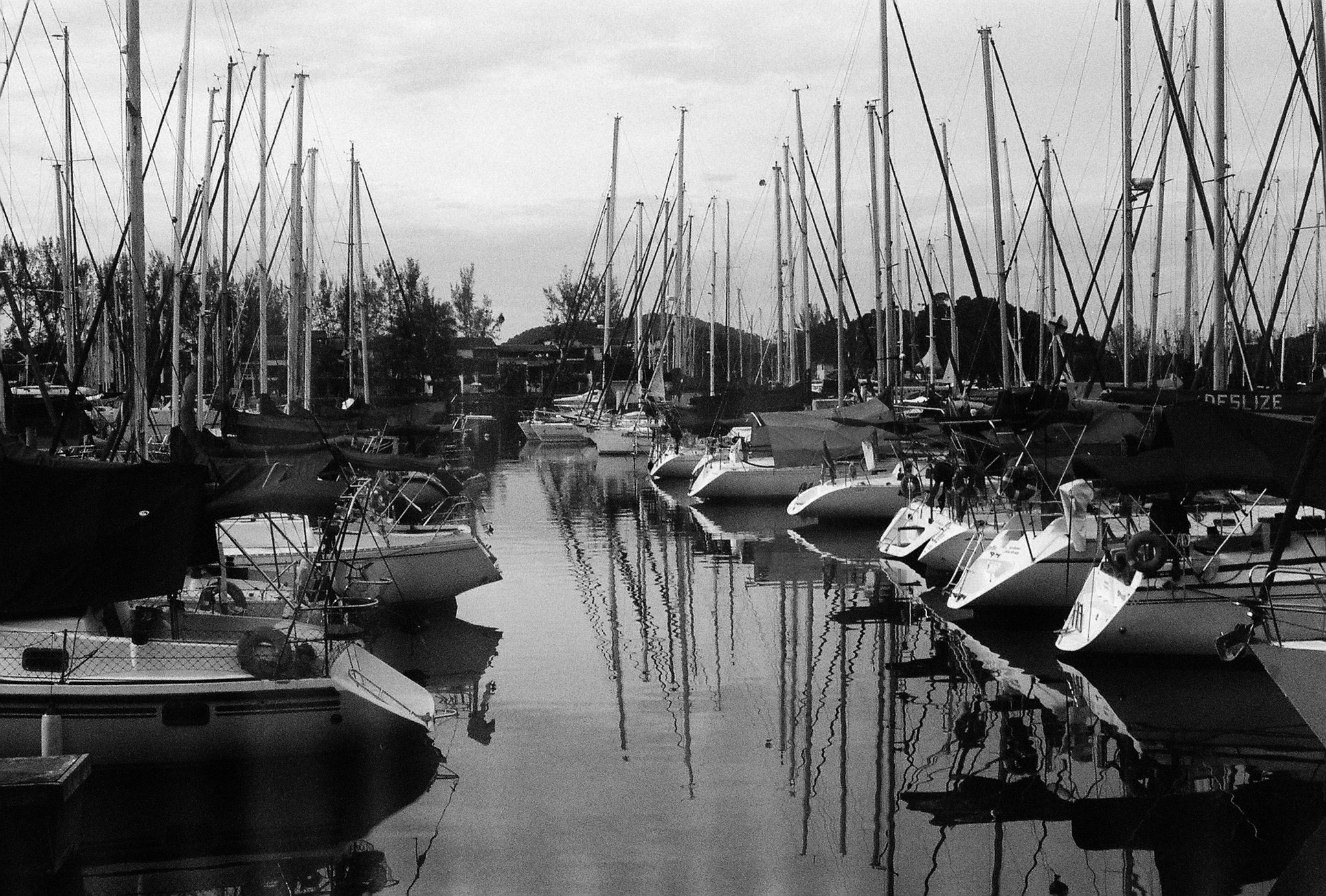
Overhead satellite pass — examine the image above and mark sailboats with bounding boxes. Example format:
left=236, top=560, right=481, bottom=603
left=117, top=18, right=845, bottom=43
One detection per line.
left=0, top=0, right=501, bottom=789
left=516, top=0, right=1325, bottom=751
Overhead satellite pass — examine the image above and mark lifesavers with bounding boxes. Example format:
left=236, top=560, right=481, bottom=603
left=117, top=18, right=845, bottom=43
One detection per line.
left=682, top=433, right=696, bottom=448
left=200, top=580, right=247, bottom=617
left=1002, top=465, right=1037, bottom=501
left=1127, top=530, right=1169, bottom=573
left=952, top=465, right=985, bottom=498
left=381, top=471, right=402, bottom=490
left=235, top=626, right=292, bottom=678
left=900, top=474, right=920, bottom=498
left=297, top=559, right=307, bottom=600
